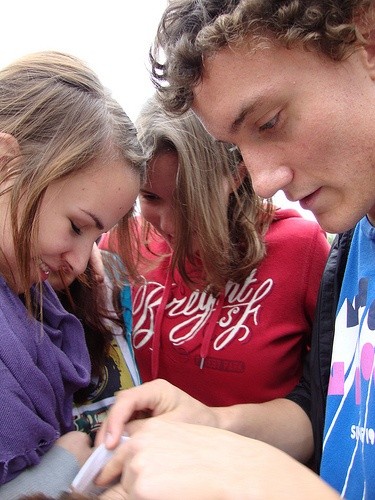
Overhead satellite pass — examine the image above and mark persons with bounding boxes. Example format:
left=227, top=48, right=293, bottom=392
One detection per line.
left=0, top=50, right=147, bottom=500
left=96, top=86, right=330, bottom=422
left=89, top=0, right=374, bottom=500
